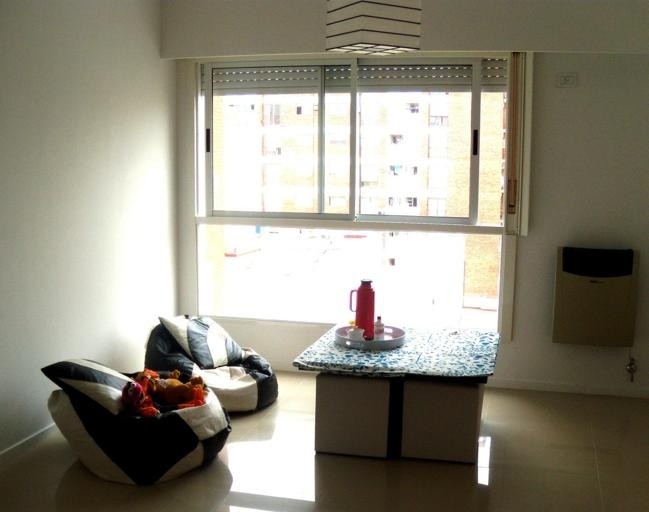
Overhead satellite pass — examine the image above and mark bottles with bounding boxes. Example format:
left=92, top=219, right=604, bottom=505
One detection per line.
left=374, top=315, right=384, bottom=333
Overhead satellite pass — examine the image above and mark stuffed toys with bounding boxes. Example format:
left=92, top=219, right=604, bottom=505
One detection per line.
left=135, top=369, right=203, bottom=401
left=121, top=375, right=159, bottom=417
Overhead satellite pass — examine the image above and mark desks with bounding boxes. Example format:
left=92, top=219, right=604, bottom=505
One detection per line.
left=294, top=316, right=497, bottom=463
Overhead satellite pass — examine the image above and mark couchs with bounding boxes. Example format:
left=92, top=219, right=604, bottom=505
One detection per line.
left=148, top=308, right=279, bottom=414
left=38, top=352, right=227, bottom=486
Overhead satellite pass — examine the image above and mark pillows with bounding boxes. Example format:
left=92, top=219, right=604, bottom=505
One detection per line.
left=41, top=356, right=137, bottom=418
left=160, top=312, right=244, bottom=370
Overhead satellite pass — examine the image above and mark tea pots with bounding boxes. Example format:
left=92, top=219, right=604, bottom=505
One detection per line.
left=345, top=325, right=364, bottom=340
left=349, top=278, right=375, bottom=341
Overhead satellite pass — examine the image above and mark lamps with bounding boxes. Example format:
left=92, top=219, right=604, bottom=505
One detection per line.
left=320, top=0, right=423, bottom=56
